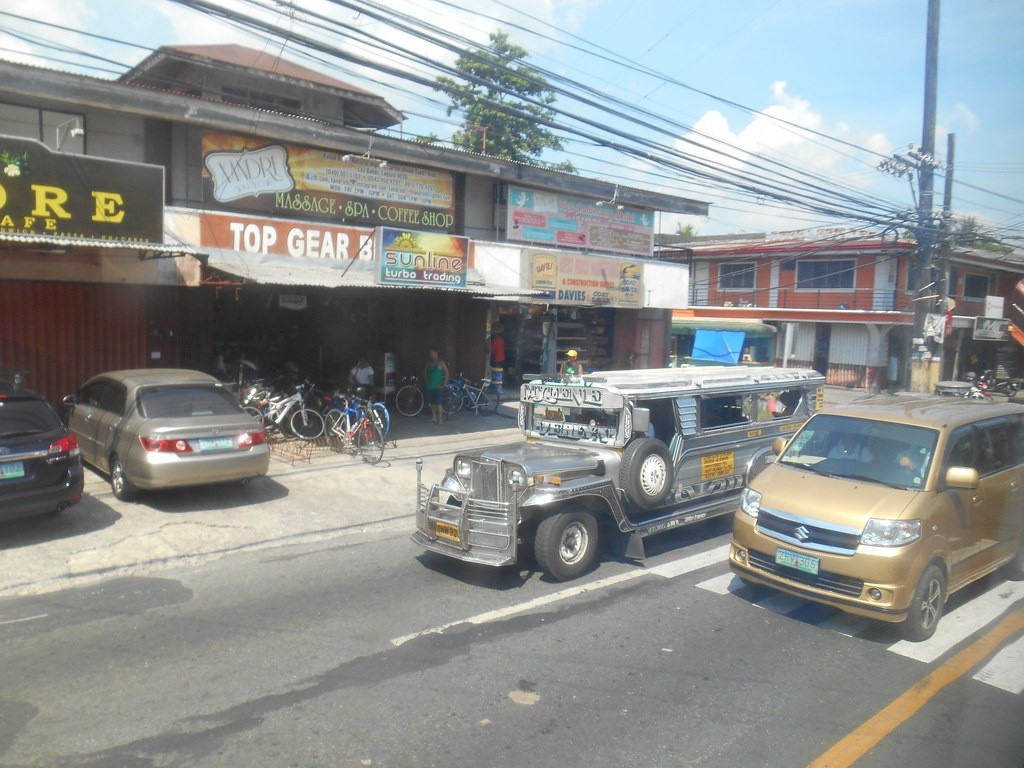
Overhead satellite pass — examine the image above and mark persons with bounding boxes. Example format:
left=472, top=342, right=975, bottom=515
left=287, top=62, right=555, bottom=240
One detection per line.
left=633, top=415, right=656, bottom=438
left=830, top=430, right=875, bottom=465
left=896, top=438, right=930, bottom=479
left=346, top=355, right=376, bottom=404
left=491, top=329, right=506, bottom=388
left=741, top=388, right=783, bottom=423
left=421, top=346, right=450, bottom=427
left=559, top=350, right=583, bottom=376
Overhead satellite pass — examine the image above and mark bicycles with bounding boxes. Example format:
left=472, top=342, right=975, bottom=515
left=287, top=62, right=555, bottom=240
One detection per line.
left=235, top=372, right=501, bottom=465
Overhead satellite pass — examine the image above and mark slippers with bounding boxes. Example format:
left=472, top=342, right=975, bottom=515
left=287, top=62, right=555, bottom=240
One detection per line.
left=423, top=419, right=443, bottom=426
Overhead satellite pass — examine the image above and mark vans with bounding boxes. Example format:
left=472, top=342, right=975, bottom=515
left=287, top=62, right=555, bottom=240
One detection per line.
left=727, top=396, right=1024, bottom=642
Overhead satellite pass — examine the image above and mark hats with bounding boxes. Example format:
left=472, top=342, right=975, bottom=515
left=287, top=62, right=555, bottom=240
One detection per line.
left=491, top=322, right=505, bottom=334
left=565, top=350, right=577, bottom=356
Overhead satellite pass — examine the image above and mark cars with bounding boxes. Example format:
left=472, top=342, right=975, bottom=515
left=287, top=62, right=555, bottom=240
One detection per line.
left=410, top=365, right=825, bottom=581
left=62, top=369, right=270, bottom=499
left=0, top=381, right=84, bottom=522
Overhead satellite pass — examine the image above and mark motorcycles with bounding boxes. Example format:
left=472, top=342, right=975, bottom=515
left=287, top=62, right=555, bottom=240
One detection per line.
left=965, top=369, right=1024, bottom=402
